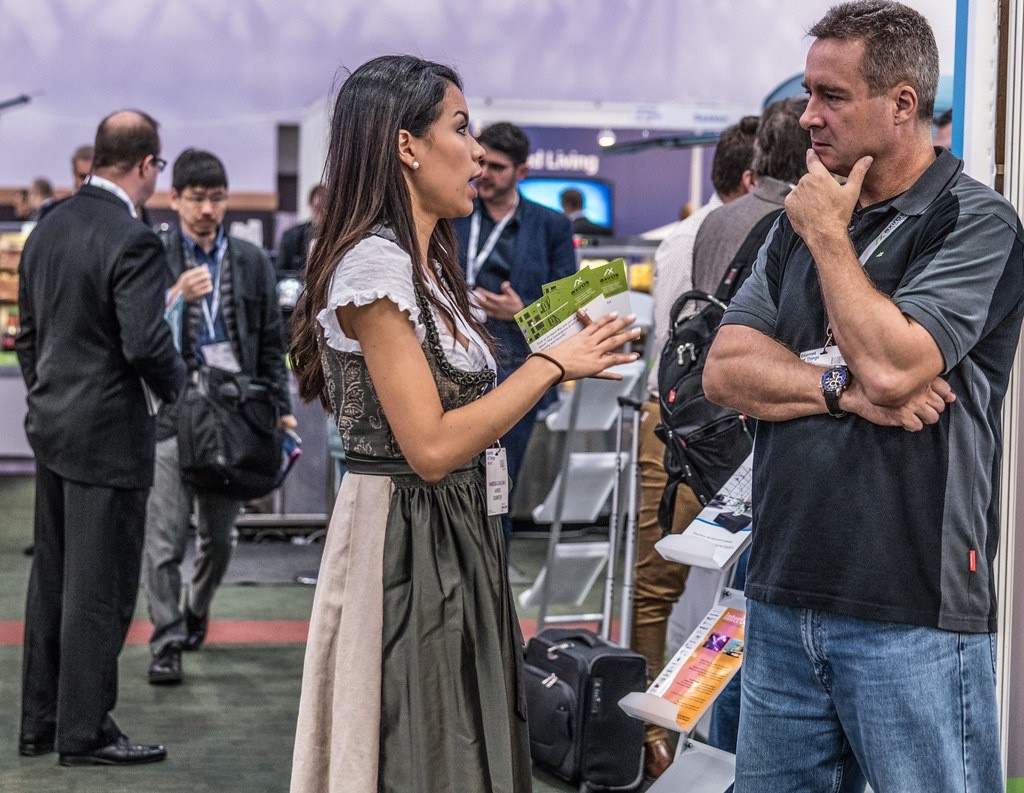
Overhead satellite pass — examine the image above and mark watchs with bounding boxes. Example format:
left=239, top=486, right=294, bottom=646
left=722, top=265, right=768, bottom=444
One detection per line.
left=819, top=364, right=853, bottom=419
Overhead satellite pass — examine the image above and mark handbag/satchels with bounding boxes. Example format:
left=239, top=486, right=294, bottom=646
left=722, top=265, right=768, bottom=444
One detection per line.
left=177, top=363, right=283, bottom=493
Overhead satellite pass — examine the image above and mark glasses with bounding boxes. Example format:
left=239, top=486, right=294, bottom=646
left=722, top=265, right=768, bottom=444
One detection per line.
left=139, top=158, right=166, bottom=170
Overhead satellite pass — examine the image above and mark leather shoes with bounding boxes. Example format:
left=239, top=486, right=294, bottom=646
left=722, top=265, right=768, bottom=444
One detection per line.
left=59, top=735, right=167, bottom=767
left=20, top=733, right=55, bottom=756
left=148, top=649, right=183, bottom=684
left=180, top=596, right=208, bottom=650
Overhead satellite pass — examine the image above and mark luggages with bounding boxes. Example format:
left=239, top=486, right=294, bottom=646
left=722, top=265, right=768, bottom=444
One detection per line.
left=523, top=397, right=648, bottom=790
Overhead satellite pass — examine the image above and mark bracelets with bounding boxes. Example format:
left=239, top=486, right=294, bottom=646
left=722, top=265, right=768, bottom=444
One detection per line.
left=526, top=352, right=565, bottom=388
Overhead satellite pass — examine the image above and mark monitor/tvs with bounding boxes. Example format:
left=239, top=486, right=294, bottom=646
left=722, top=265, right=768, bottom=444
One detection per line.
left=516, top=175, right=611, bottom=229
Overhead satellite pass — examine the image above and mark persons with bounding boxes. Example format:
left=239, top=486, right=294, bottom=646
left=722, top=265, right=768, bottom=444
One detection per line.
left=8, top=105, right=300, bottom=768
left=631, top=93, right=813, bottom=782
left=444, top=119, right=575, bottom=568
left=287, top=49, right=648, bottom=793
left=560, top=189, right=616, bottom=238
left=276, top=184, right=327, bottom=284
left=932, top=107, right=954, bottom=151
left=703, top=0, right=1024, bottom=792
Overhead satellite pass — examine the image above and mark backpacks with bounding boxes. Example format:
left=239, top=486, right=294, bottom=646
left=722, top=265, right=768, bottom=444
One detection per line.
left=653, top=208, right=782, bottom=539
